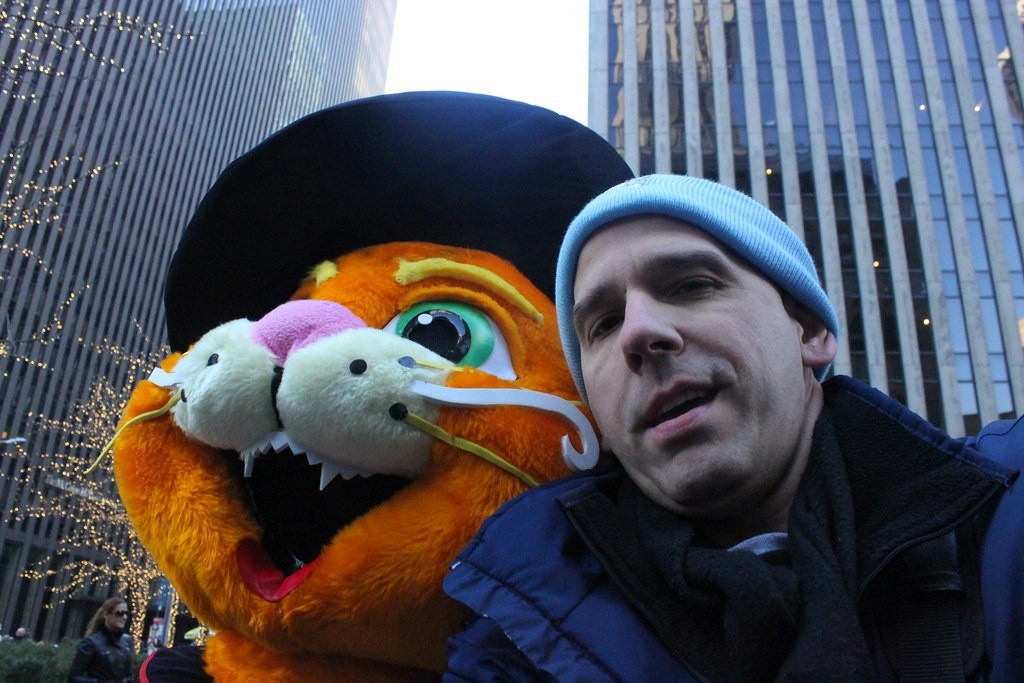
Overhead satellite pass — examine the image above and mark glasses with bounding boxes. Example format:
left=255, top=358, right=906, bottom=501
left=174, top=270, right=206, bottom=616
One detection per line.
left=110, top=611, right=130, bottom=616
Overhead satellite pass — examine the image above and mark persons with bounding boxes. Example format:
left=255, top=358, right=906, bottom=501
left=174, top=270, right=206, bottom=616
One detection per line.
left=12, top=626, right=30, bottom=641
left=433, top=174, right=1024, bottom=683
left=66, top=595, right=135, bottom=683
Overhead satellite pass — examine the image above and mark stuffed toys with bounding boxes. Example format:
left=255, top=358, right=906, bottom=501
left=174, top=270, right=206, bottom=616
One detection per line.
left=112, top=89, right=640, bottom=682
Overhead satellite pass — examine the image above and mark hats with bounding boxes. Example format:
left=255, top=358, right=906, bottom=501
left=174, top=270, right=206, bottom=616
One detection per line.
left=555, top=174, right=839, bottom=415
